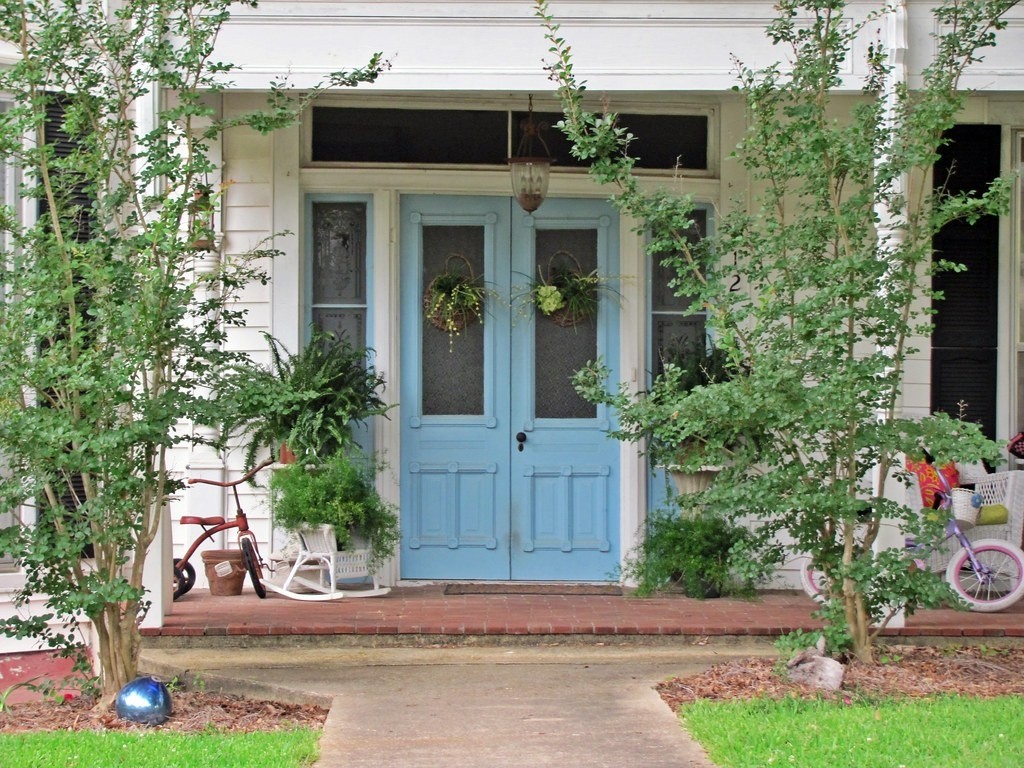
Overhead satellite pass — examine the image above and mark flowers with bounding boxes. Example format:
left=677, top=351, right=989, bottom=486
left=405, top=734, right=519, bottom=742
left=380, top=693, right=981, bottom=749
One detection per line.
left=418, top=259, right=510, bottom=355
left=508, top=265, right=646, bottom=337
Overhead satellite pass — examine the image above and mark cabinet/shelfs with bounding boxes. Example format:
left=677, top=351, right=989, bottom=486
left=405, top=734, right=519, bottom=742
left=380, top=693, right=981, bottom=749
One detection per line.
left=268, top=464, right=333, bottom=581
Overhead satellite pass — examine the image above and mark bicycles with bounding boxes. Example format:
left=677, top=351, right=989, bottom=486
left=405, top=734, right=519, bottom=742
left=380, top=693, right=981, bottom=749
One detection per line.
left=799, top=445, right=1024, bottom=613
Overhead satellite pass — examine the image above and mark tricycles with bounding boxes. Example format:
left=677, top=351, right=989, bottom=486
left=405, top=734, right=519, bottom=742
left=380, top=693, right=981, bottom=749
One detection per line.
left=172, top=454, right=278, bottom=603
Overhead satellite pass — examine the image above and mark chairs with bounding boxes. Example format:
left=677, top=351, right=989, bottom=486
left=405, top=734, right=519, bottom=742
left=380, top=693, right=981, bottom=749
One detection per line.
left=905, top=415, right=1024, bottom=582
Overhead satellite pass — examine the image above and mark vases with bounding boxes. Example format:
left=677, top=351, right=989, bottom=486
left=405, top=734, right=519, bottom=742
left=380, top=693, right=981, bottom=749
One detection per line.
left=201, top=549, right=248, bottom=596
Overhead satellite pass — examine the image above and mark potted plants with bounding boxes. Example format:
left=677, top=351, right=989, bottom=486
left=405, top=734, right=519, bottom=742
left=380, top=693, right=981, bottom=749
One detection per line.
left=605, top=508, right=751, bottom=601
left=256, top=446, right=402, bottom=585
left=631, top=335, right=769, bottom=466
left=216, top=321, right=401, bottom=489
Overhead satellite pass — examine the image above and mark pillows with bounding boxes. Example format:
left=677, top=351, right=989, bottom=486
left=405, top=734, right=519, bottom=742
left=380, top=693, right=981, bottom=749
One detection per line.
left=905, top=453, right=959, bottom=508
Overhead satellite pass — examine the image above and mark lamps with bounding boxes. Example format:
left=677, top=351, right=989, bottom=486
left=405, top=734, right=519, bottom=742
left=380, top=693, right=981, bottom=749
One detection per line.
left=508, top=93, right=558, bottom=216
left=185, top=155, right=217, bottom=253
